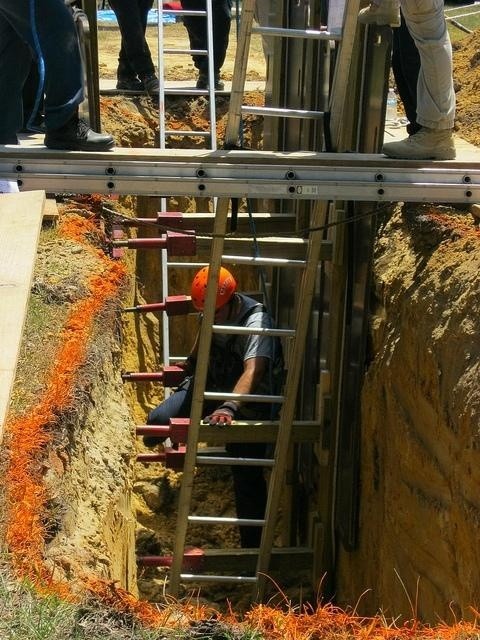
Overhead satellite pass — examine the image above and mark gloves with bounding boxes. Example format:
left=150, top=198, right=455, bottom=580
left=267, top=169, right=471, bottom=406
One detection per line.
left=203, top=402, right=238, bottom=428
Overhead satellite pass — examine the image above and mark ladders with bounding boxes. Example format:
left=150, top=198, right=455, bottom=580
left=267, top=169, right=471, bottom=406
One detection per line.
left=156, top=0, right=360, bottom=609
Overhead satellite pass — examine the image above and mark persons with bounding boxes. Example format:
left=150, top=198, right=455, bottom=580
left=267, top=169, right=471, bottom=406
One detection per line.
left=357, top=0, right=458, bottom=161
left=141, top=265, right=288, bottom=599
left=107, top=0, right=162, bottom=91
left=1, top=0, right=116, bottom=152
left=20, top=0, right=57, bottom=136
left=386, top=0, right=431, bottom=142
left=179, top=0, right=233, bottom=90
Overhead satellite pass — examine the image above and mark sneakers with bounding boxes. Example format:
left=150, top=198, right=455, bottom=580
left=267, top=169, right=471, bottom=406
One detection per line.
left=44, top=120, right=114, bottom=151
left=382, top=128, right=456, bottom=160
left=358, top=0, right=401, bottom=27
left=196, top=73, right=225, bottom=89
left=116, top=74, right=159, bottom=91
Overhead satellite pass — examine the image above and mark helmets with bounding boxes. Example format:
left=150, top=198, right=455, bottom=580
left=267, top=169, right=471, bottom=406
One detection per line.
left=191, top=266, right=236, bottom=311
